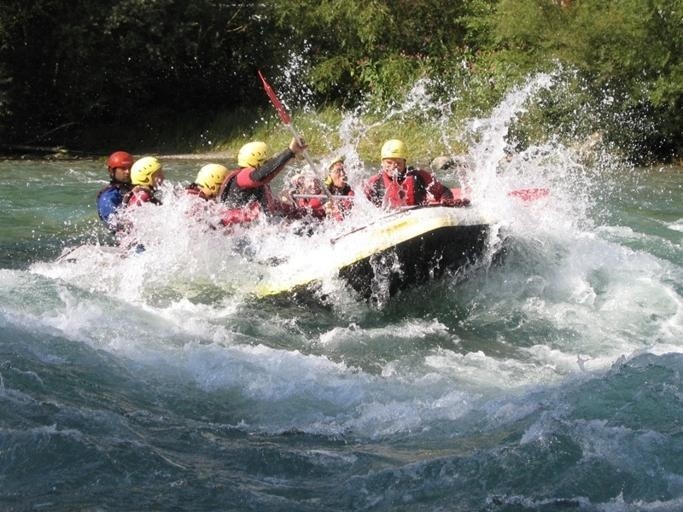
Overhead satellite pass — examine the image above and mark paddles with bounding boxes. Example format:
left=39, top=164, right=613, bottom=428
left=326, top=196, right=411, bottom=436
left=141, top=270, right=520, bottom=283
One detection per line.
left=291, top=189, right=549, bottom=206
left=258, top=70, right=343, bottom=222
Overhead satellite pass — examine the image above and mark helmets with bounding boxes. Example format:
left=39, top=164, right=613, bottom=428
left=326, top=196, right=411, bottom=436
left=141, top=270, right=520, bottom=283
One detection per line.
left=238, top=142, right=272, bottom=168
left=106, top=151, right=161, bottom=185
left=381, top=140, right=408, bottom=168
left=194, top=163, right=230, bottom=197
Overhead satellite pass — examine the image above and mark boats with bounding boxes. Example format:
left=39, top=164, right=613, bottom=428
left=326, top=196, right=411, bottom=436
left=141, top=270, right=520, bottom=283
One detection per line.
left=253, top=203, right=506, bottom=311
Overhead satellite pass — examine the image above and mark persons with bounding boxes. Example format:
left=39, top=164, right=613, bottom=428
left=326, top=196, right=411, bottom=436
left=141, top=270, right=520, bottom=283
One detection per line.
left=93, top=150, right=142, bottom=254
left=178, top=161, right=229, bottom=230
left=360, top=138, right=455, bottom=209
left=122, top=155, right=165, bottom=209
left=215, top=133, right=339, bottom=238
left=319, top=152, right=355, bottom=221
left=280, top=170, right=324, bottom=210
